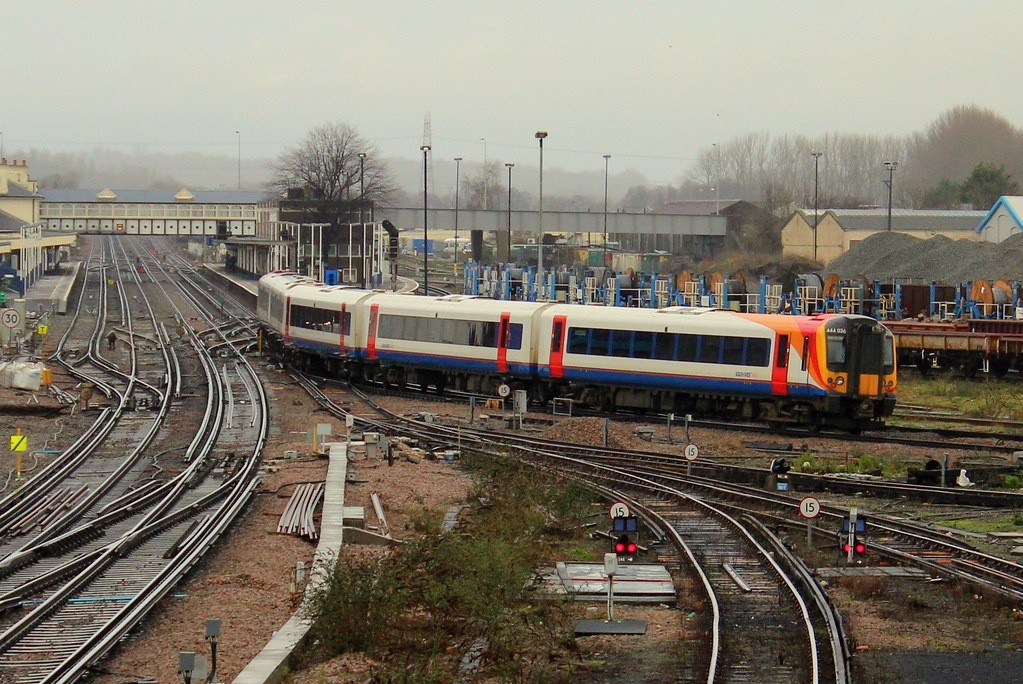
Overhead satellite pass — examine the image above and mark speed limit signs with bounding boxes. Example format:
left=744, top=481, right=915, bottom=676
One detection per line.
left=2, top=309, right=20, bottom=328
left=799, top=496, right=820, bottom=518
left=609, top=502, right=629, bottom=521
left=684, top=444, right=699, bottom=460
left=498, top=384, right=510, bottom=397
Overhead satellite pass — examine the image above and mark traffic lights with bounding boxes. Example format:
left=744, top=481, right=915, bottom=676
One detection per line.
left=614, top=536, right=637, bottom=557
left=841, top=537, right=865, bottom=559
left=770, top=458, right=792, bottom=473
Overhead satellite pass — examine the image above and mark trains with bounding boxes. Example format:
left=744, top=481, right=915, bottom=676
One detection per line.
left=258, top=269, right=897, bottom=436
left=443, top=237, right=471, bottom=254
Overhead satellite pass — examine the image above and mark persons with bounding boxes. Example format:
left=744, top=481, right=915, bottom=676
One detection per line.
left=226, top=252, right=230, bottom=267
left=106, top=328, right=118, bottom=351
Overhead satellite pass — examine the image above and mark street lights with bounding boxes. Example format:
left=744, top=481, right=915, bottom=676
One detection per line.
left=357, top=152, right=367, bottom=290
left=535, top=130, right=548, bottom=302
left=454, top=157, right=462, bottom=262
left=712, top=141, right=720, bottom=215
left=811, top=151, right=822, bottom=261
left=884, top=161, right=899, bottom=230
left=479, top=136, right=486, bottom=210
left=505, top=162, right=514, bottom=263
left=420, top=145, right=431, bottom=296
left=235, top=130, right=240, bottom=190
left=603, top=154, right=612, bottom=267
